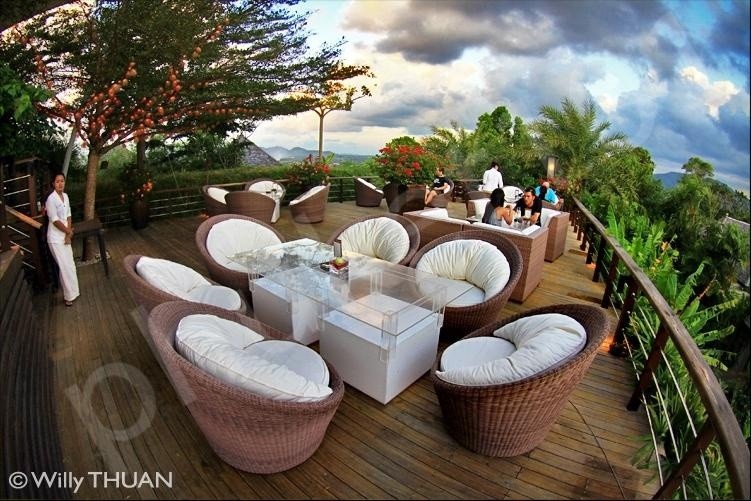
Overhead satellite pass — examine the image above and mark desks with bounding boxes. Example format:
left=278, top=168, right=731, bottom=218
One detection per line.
left=68, top=218, right=112, bottom=285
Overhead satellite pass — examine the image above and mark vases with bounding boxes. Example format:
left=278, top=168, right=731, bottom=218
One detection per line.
left=128, top=200, right=147, bottom=229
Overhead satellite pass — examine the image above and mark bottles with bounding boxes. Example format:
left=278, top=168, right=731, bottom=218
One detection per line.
left=451, top=185, right=458, bottom=202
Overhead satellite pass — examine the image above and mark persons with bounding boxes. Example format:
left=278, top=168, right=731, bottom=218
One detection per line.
left=512, top=185, right=543, bottom=228
left=424, top=166, right=449, bottom=206
left=482, top=162, right=503, bottom=192
left=534, top=181, right=559, bottom=207
left=481, top=187, right=514, bottom=227
left=43, top=172, right=80, bottom=307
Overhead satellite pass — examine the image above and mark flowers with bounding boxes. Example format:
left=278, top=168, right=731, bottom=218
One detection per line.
left=376, top=137, right=449, bottom=184
left=117, top=163, right=155, bottom=204
left=537, top=174, right=580, bottom=203
left=290, top=155, right=335, bottom=188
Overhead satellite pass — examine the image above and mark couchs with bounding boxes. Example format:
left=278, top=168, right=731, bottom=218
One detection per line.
left=433, top=306, right=614, bottom=463
left=195, top=213, right=288, bottom=284
left=410, top=227, right=530, bottom=335
left=187, top=174, right=333, bottom=226
left=353, top=177, right=571, bottom=306
left=330, top=211, right=422, bottom=266
left=146, top=302, right=348, bottom=476
left=126, top=255, right=248, bottom=318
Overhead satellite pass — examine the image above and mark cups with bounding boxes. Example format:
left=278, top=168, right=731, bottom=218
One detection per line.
left=513, top=205, right=540, bottom=229
